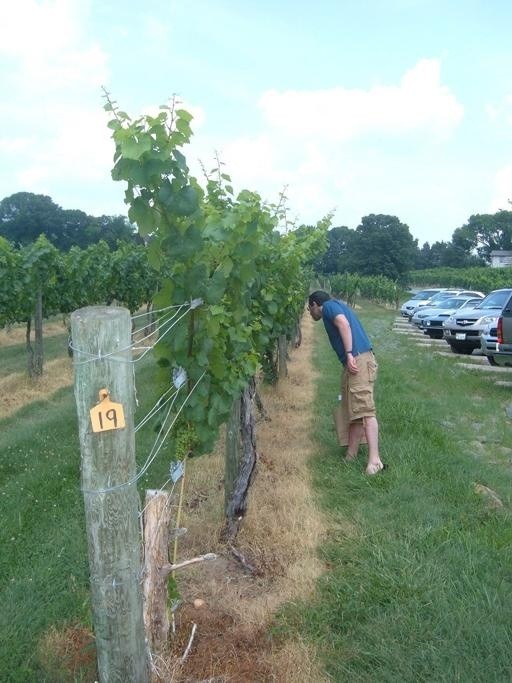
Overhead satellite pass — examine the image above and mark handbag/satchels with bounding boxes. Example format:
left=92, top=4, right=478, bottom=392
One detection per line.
left=333, top=407, right=368, bottom=446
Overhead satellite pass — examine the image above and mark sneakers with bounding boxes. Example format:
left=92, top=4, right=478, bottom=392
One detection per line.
left=366, top=458, right=383, bottom=474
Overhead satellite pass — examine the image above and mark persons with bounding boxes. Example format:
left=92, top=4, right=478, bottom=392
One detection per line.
left=308, top=291, right=388, bottom=478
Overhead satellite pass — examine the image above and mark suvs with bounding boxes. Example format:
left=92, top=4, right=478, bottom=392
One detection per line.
left=441, top=288, right=512, bottom=352
left=493, top=293, right=512, bottom=365
left=481, top=319, right=498, bottom=366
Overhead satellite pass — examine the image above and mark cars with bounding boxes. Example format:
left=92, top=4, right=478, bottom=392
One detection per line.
left=401, top=286, right=497, bottom=338
